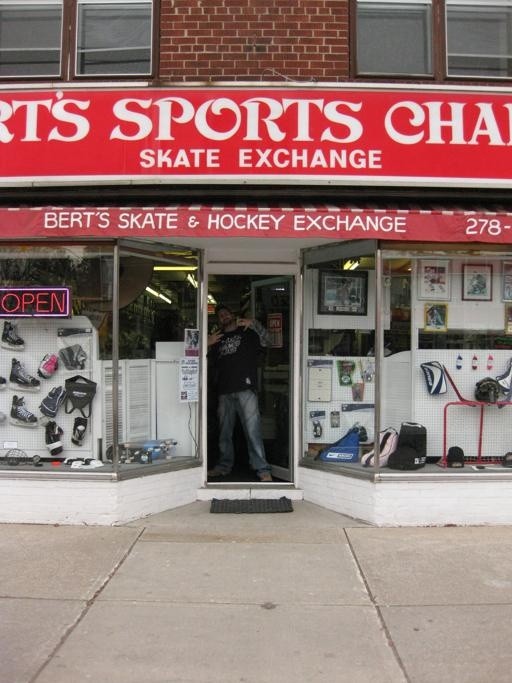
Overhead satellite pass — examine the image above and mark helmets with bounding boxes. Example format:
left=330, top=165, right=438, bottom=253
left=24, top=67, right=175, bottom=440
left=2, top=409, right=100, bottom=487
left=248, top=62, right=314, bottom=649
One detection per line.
left=474, top=377, right=500, bottom=402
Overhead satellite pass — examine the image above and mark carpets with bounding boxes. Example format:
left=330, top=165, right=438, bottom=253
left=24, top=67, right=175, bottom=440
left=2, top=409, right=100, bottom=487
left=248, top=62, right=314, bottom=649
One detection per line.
left=210, top=496, right=293, bottom=513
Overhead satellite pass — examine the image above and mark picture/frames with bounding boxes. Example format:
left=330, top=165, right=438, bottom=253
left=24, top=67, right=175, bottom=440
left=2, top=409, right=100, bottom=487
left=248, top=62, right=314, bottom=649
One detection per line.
left=505, top=302, right=512, bottom=337
left=424, top=303, right=448, bottom=333
left=318, top=268, right=368, bottom=316
left=417, top=258, right=451, bottom=301
left=501, top=260, right=512, bottom=302
left=462, top=263, right=492, bottom=301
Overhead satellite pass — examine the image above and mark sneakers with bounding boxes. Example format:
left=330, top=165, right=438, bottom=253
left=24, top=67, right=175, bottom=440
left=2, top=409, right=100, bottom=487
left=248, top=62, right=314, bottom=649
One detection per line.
left=208, top=468, right=232, bottom=478
left=259, top=471, right=272, bottom=482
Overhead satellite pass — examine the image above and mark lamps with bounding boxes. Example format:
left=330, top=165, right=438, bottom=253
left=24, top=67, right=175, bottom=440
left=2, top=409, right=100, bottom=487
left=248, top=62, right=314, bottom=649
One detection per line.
left=343, top=257, right=361, bottom=270
left=159, top=291, right=173, bottom=305
left=145, top=283, right=160, bottom=297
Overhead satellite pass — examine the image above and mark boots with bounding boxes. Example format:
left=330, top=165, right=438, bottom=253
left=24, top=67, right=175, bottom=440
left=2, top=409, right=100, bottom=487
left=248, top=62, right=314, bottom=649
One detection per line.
left=2, top=321, right=26, bottom=345
left=11, top=395, right=39, bottom=423
left=9, top=358, right=42, bottom=385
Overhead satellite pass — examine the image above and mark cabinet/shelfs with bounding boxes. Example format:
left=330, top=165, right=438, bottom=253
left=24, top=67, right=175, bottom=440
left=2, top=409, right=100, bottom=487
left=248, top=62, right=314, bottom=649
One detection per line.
left=0, top=315, right=99, bottom=460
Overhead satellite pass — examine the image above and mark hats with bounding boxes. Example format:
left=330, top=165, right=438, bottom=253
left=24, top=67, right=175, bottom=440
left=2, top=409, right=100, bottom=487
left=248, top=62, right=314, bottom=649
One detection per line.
left=214, top=303, right=230, bottom=314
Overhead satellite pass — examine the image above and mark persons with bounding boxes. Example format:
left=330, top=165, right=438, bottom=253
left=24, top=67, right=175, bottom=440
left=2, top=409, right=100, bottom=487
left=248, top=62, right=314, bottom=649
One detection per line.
left=426, top=267, right=445, bottom=293
left=337, top=279, right=352, bottom=304
left=208, top=302, right=274, bottom=482
left=427, top=307, right=444, bottom=325
left=469, top=269, right=486, bottom=294
